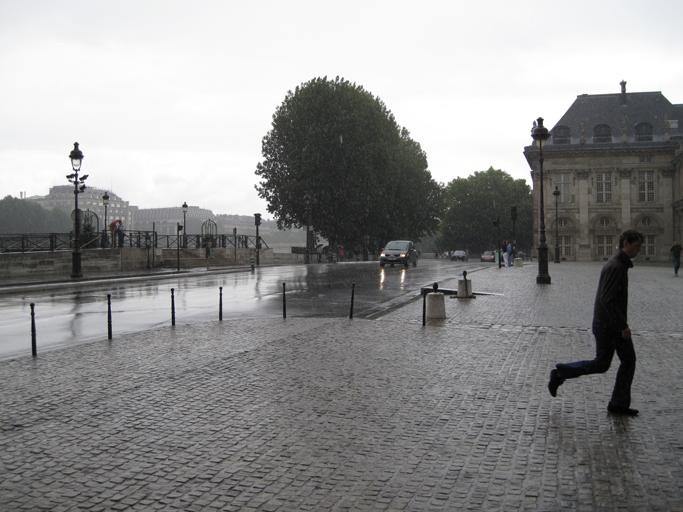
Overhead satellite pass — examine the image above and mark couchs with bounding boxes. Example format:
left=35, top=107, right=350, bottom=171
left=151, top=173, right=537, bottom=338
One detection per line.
left=254, top=213, right=261, bottom=226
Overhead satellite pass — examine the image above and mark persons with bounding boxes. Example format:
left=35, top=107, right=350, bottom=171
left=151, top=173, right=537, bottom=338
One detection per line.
left=670, top=240, right=683, bottom=275
left=548, top=230, right=644, bottom=417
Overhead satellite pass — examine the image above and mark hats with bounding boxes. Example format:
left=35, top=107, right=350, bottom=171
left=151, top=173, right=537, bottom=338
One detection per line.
left=450, top=250, right=468, bottom=262
left=480, top=250, right=496, bottom=262
left=377, top=240, right=418, bottom=268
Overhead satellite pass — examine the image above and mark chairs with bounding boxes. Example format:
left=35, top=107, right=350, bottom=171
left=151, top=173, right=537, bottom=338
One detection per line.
left=605, top=400, right=640, bottom=417
left=547, top=367, right=564, bottom=397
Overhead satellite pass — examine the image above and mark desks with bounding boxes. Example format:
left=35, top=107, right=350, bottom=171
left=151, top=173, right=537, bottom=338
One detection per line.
left=99, top=191, right=112, bottom=249
left=180, top=201, right=189, bottom=248
left=65, top=141, right=89, bottom=279
left=551, top=185, right=561, bottom=263
left=530, top=116, right=551, bottom=285
